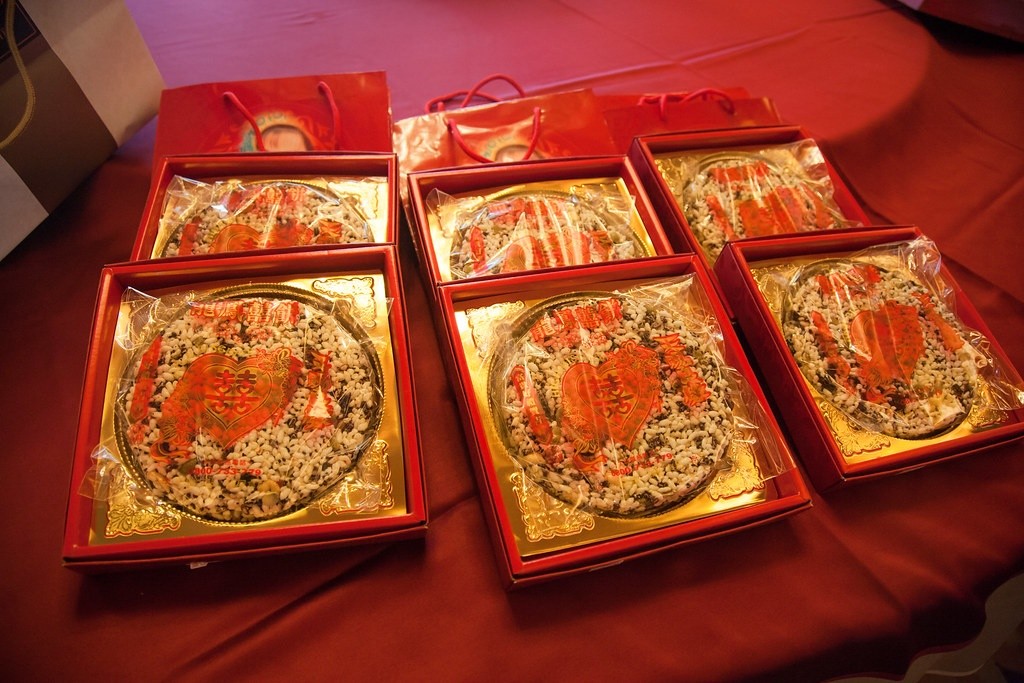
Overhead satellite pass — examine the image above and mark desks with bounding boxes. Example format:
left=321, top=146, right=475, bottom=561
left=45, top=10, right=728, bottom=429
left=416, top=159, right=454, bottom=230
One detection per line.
left=0, top=0, right=1024, bottom=682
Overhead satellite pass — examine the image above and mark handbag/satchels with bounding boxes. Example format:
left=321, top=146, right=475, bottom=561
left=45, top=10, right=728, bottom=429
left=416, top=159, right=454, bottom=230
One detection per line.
left=596, top=91, right=781, bottom=156
left=389, top=75, right=625, bottom=252
left=152, top=71, right=393, bottom=162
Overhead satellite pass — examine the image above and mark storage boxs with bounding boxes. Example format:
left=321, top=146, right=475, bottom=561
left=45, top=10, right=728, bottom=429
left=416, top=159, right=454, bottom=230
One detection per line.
left=62, top=150, right=1023, bottom=593
